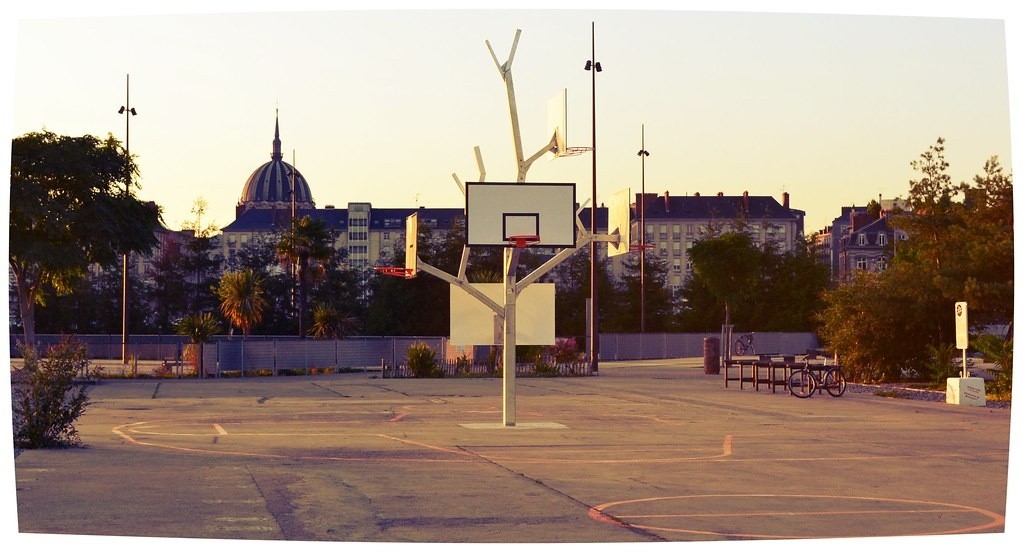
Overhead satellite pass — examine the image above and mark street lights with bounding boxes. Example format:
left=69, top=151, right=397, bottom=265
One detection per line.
left=117, top=73, right=137, bottom=366
left=286, top=147, right=301, bottom=339
left=584, top=21, right=602, bottom=373
left=636, top=123, right=650, bottom=332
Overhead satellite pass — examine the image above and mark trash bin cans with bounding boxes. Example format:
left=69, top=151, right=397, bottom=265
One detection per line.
left=703, top=336, right=721, bottom=375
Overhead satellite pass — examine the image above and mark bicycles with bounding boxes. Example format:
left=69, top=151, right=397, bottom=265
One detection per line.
left=734, top=332, right=757, bottom=356
left=787, top=353, right=847, bottom=399
